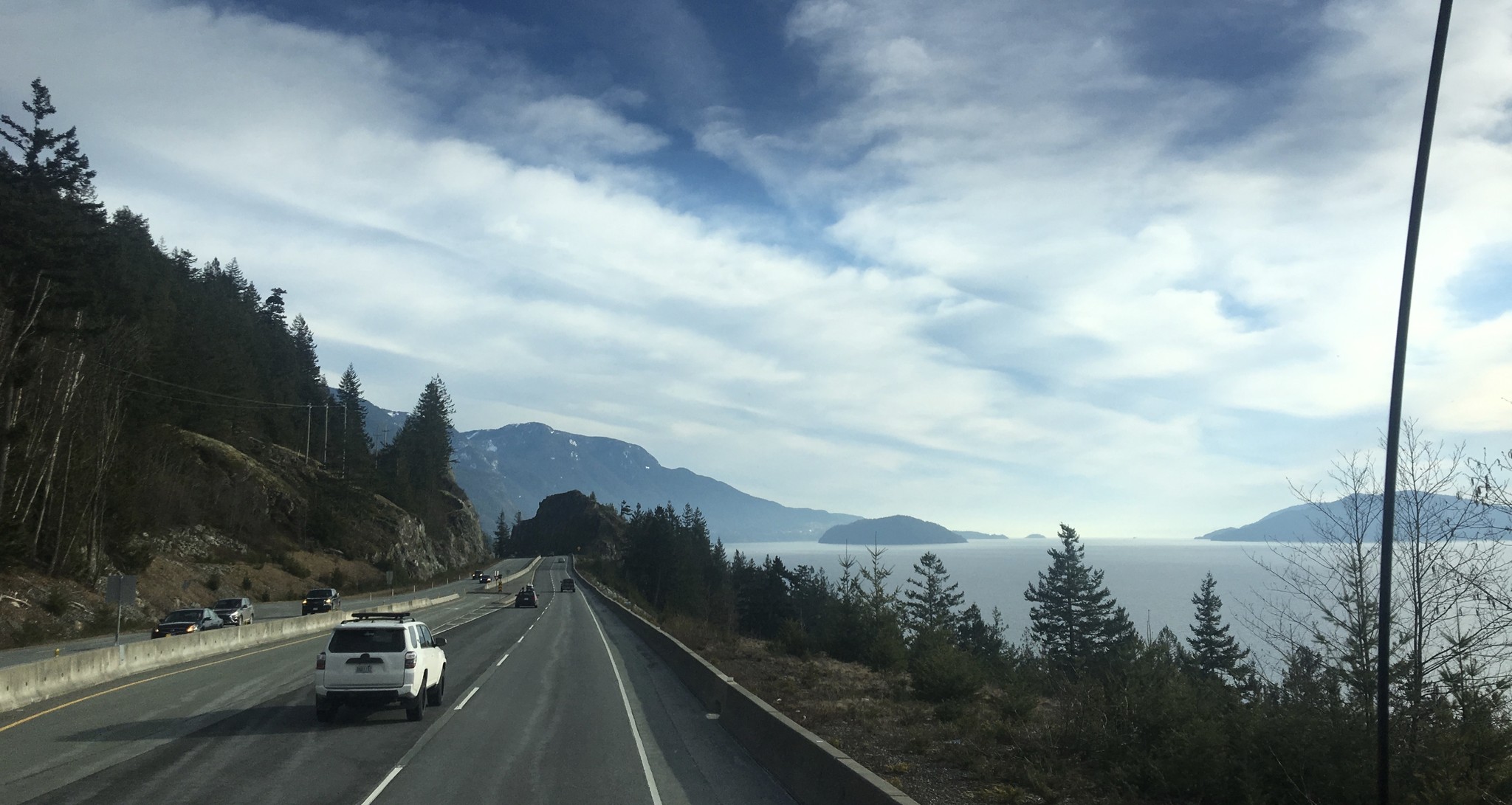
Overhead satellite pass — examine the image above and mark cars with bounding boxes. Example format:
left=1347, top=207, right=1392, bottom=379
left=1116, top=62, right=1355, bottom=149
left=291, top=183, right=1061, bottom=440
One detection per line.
left=150, top=608, right=225, bottom=640
left=558, top=558, right=565, bottom=563
left=472, top=570, right=485, bottom=579
left=302, top=588, right=341, bottom=616
left=560, top=579, right=577, bottom=593
left=479, top=575, right=493, bottom=583
left=514, top=590, right=539, bottom=608
left=211, top=598, right=255, bottom=626
left=548, top=551, right=554, bottom=556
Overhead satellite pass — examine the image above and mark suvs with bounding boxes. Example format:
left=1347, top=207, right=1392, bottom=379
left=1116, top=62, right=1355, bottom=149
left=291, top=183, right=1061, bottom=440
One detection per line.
left=315, top=613, right=448, bottom=721
